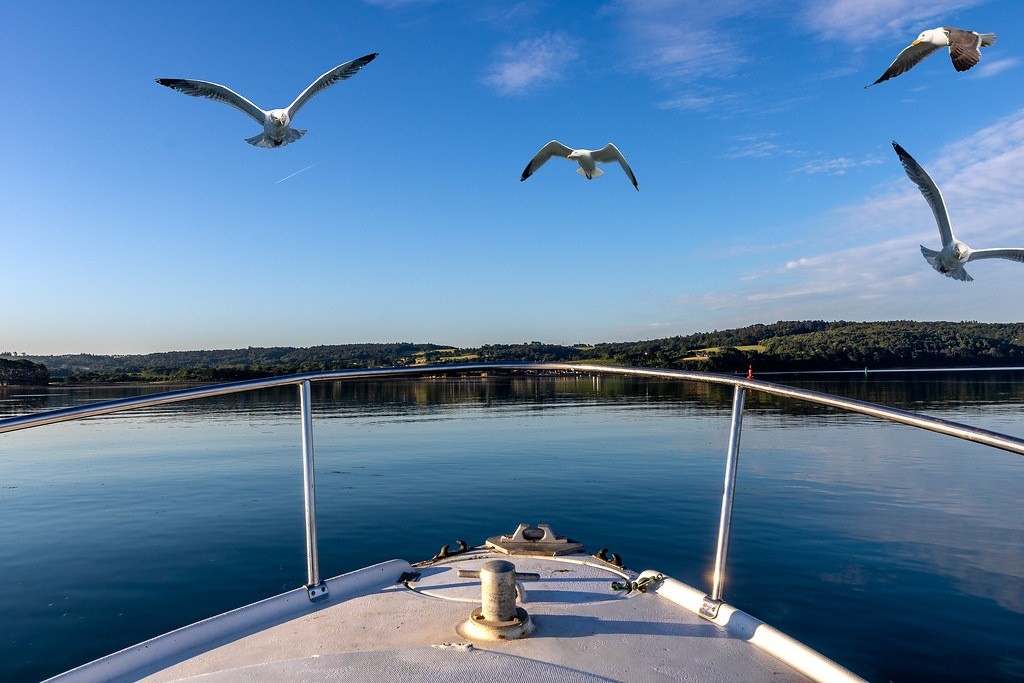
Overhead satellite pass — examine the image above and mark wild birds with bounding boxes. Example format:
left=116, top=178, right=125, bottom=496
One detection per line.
left=153, top=52, right=379, bottom=149
left=892, top=141, right=1024, bottom=281
left=865, top=27, right=996, bottom=89
left=519, top=139, right=638, bottom=192
